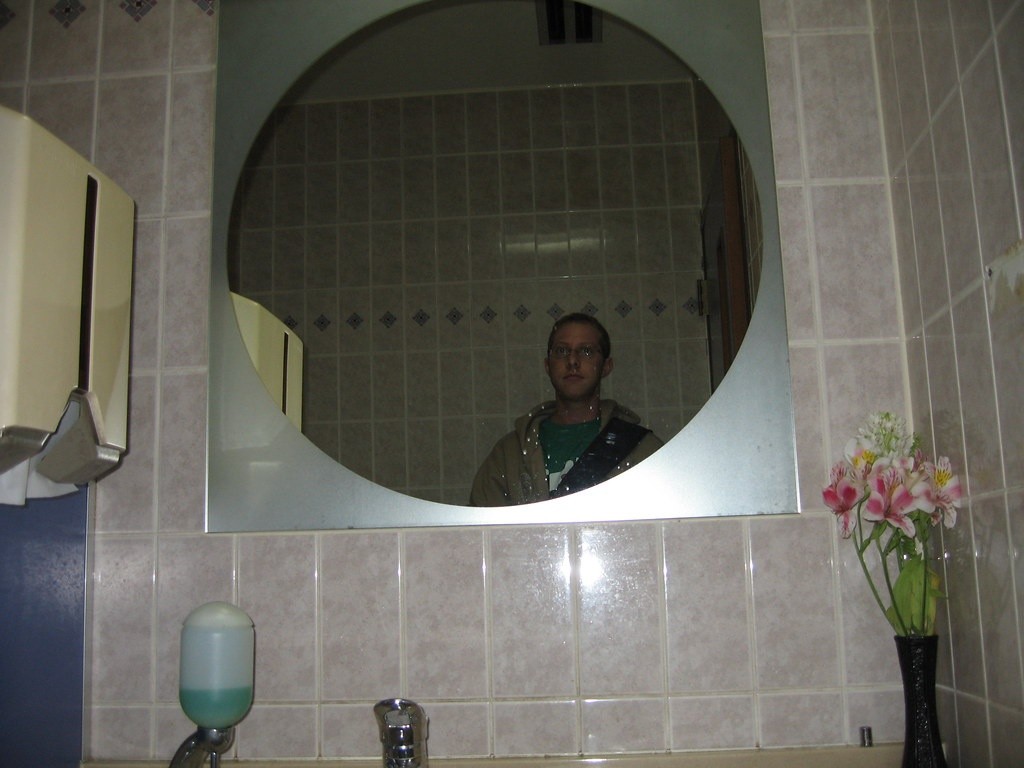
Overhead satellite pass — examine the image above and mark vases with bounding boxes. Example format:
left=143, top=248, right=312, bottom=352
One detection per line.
left=891, top=632, right=950, bottom=767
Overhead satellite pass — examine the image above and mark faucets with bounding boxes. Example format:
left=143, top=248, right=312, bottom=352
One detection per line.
left=372, top=695, right=431, bottom=768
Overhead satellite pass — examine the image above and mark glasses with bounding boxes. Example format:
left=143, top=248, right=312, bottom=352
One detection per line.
left=549, top=343, right=602, bottom=360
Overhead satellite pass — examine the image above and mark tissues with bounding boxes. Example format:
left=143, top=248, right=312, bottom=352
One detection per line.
left=0, top=102, right=139, bottom=509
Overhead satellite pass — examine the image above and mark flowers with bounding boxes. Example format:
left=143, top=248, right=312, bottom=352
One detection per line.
left=818, top=407, right=964, bottom=639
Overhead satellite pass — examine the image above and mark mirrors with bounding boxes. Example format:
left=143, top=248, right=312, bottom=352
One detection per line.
left=202, top=0, right=803, bottom=531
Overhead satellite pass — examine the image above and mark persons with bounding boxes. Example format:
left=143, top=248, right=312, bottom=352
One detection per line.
left=468, top=312, right=667, bottom=506
